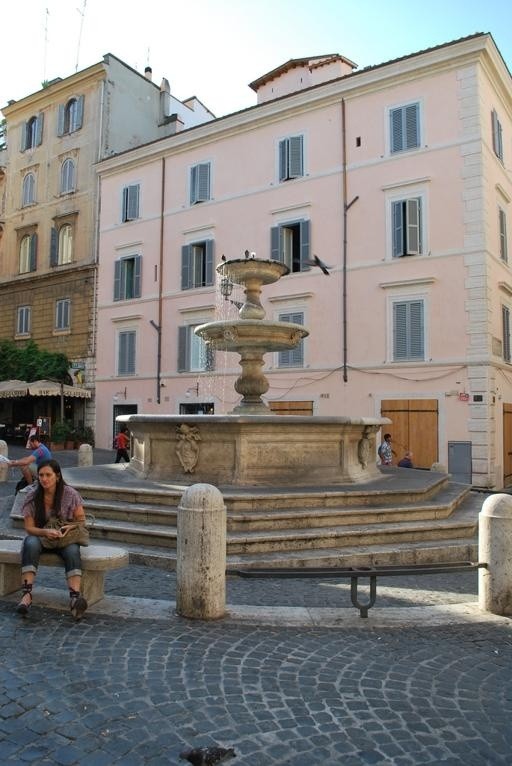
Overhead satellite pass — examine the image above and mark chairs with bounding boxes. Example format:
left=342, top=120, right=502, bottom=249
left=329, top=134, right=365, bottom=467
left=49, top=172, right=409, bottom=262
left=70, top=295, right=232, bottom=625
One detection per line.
left=0, top=422, right=33, bottom=446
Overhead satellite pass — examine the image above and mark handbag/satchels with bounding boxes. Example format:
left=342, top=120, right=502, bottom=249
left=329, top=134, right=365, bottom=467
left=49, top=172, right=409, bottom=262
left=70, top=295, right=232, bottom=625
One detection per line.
left=39, top=518, right=90, bottom=549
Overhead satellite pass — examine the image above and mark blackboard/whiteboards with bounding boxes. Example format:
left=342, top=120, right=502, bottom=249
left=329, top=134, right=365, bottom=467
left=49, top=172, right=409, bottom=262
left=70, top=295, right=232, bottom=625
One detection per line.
left=39, top=416, right=51, bottom=435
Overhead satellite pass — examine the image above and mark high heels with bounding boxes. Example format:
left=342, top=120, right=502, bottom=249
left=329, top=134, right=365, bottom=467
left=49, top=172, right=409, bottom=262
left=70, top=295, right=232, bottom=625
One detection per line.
left=69, top=586, right=88, bottom=622
left=16, top=580, right=33, bottom=618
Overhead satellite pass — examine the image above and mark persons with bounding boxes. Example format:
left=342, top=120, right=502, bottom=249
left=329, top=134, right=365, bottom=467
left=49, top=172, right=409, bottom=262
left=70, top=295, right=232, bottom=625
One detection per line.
left=379, top=433, right=397, bottom=465
left=0, top=440, right=15, bottom=480
left=396, top=451, right=414, bottom=469
left=114, top=426, right=130, bottom=463
left=9, top=436, right=52, bottom=487
left=16, top=457, right=91, bottom=621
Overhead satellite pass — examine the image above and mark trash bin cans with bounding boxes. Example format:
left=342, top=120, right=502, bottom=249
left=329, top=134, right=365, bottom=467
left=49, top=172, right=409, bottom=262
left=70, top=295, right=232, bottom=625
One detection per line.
left=398, top=458, right=412, bottom=468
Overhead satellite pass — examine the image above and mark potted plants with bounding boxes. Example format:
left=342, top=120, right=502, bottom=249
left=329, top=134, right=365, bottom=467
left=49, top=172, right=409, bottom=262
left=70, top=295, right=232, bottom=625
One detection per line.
left=45, top=417, right=94, bottom=451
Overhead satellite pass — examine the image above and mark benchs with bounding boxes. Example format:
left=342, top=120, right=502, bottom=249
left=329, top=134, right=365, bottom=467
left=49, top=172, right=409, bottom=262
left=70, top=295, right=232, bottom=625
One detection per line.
left=0, top=539, right=130, bottom=611
